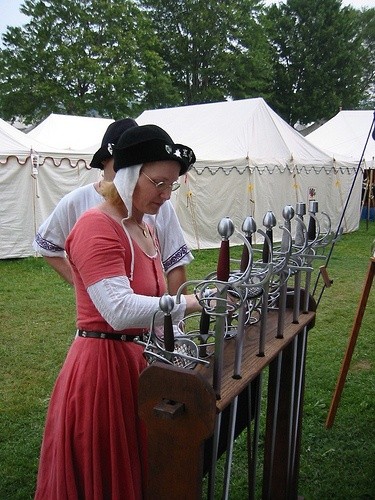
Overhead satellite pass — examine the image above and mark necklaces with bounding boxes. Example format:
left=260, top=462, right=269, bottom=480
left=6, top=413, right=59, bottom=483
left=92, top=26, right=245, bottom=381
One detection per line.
left=120, top=205, right=151, bottom=238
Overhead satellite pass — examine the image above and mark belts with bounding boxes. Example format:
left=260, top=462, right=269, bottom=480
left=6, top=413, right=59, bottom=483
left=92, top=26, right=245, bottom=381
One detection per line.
left=78, top=330, right=148, bottom=342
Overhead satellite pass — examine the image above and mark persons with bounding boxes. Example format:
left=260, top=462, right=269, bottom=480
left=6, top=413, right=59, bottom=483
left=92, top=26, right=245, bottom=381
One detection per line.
left=35, top=126, right=240, bottom=500
left=33, top=117, right=194, bottom=329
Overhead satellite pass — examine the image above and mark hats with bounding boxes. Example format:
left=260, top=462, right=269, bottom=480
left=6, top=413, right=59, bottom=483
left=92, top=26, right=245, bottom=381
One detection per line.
left=90, top=118, right=139, bottom=169
left=113, top=125, right=196, bottom=176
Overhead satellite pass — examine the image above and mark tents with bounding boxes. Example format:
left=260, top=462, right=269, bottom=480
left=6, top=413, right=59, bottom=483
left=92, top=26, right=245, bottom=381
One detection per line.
left=0, top=97, right=373, bottom=259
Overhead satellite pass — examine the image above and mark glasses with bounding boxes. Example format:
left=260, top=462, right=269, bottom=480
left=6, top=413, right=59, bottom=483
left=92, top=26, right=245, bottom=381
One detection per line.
left=140, top=169, right=181, bottom=191
